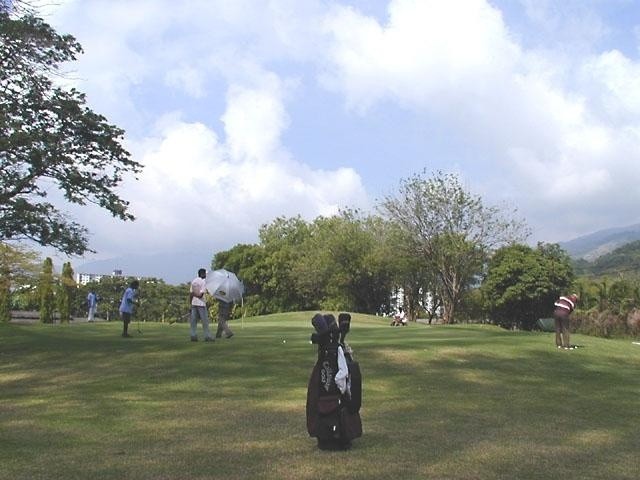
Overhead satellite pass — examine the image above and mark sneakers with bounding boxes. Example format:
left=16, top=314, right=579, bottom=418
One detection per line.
left=122, top=333, right=131, bottom=337
left=191, top=334, right=234, bottom=342
left=558, top=345, right=575, bottom=350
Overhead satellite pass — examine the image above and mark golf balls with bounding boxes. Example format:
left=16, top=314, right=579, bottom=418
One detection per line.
left=283, top=340, right=286, bottom=343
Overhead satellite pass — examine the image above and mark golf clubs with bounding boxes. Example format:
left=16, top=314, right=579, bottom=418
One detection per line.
left=312, top=313, right=351, bottom=346
left=137, top=306, right=142, bottom=333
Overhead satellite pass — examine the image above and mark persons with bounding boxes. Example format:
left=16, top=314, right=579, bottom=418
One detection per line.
left=213, top=297, right=234, bottom=338
left=189, top=268, right=215, bottom=342
left=87, top=287, right=99, bottom=322
left=391, top=306, right=408, bottom=327
left=118, top=280, right=141, bottom=337
left=553, top=292, right=579, bottom=349
